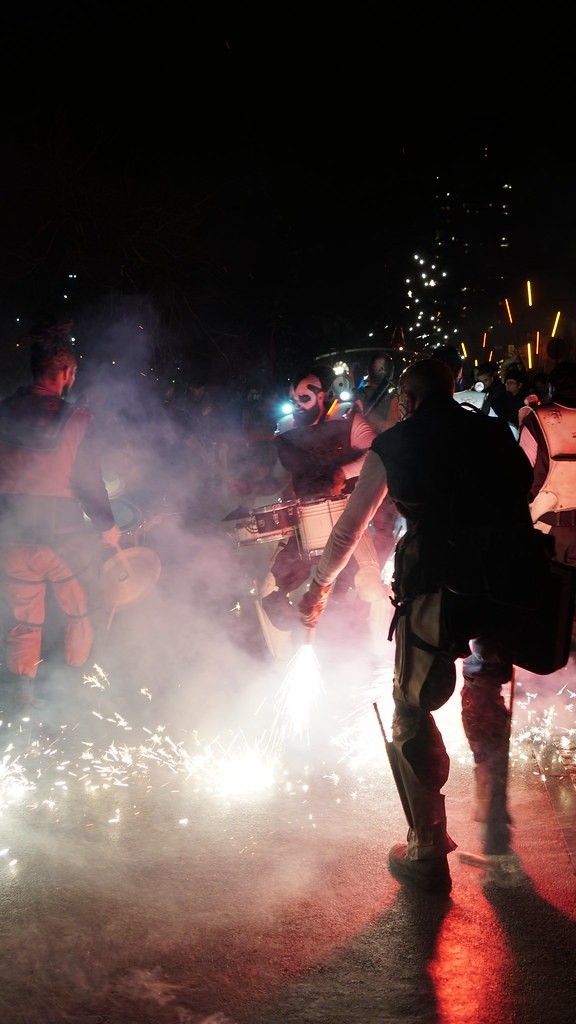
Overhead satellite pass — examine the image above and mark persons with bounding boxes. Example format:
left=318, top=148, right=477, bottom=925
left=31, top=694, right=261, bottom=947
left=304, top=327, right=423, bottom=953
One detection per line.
left=0, top=339, right=119, bottom=718
left=257, top=346, right=576, bottom=668
left=295, top=360, right=533, bottom=893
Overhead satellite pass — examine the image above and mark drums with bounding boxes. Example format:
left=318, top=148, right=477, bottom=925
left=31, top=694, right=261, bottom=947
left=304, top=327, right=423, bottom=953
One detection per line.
left=295, top=490, right=359, bottom=564
left=252, top=491, right=331, bottom=541
left=228, top=506, right=299, bottom=548
left=84, top=497, right=146, bottom=556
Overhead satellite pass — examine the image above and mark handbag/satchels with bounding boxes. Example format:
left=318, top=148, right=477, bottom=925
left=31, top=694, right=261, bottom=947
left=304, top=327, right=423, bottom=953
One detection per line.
left=504, top=524, right=576, bottom=674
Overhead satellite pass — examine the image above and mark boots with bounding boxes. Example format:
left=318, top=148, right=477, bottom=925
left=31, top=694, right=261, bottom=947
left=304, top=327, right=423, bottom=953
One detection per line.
left=13, top=676, right=48, bottom=711
left=476, top=819, right=511, bottom=855
left=64, top=663, right=103, bottom=701
left=389, top=826, right=452, bottom=899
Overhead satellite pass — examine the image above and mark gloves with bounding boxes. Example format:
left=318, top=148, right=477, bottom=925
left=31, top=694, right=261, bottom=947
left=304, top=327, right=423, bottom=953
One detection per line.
left=298, top=581, right=332, bottom=629
left=325, top=468, right=344, bottom=497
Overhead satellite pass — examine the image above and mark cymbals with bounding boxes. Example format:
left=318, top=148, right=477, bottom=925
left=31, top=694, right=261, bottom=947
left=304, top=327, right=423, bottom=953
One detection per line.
left=100, top=544, right=162, bottom=611
